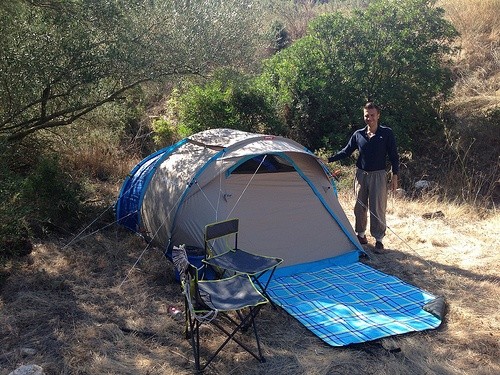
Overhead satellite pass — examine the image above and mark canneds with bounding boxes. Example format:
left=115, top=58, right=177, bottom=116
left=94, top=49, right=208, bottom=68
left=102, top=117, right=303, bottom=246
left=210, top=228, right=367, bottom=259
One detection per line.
left=167, top=306, right=183, bottom=320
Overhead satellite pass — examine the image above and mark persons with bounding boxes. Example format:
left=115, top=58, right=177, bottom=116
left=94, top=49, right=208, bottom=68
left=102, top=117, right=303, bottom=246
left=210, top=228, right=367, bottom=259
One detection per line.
left=318, top=100, right=398, bottom=253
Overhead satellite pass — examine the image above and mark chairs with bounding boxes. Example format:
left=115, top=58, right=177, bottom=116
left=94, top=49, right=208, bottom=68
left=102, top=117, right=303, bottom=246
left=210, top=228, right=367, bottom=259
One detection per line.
left=172, top=218, right=283, bottom=375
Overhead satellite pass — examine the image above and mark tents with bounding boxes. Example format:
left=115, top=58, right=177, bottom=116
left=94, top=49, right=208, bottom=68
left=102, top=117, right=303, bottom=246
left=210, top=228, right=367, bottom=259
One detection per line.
left=116, top=128, right=368, bottom=277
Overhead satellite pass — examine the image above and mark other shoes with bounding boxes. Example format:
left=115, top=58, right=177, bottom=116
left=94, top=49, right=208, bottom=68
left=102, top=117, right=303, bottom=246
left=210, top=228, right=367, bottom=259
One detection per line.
left=375, top=241, right=384, bottom=254
left=356, top=234, right=368, bottom=245
